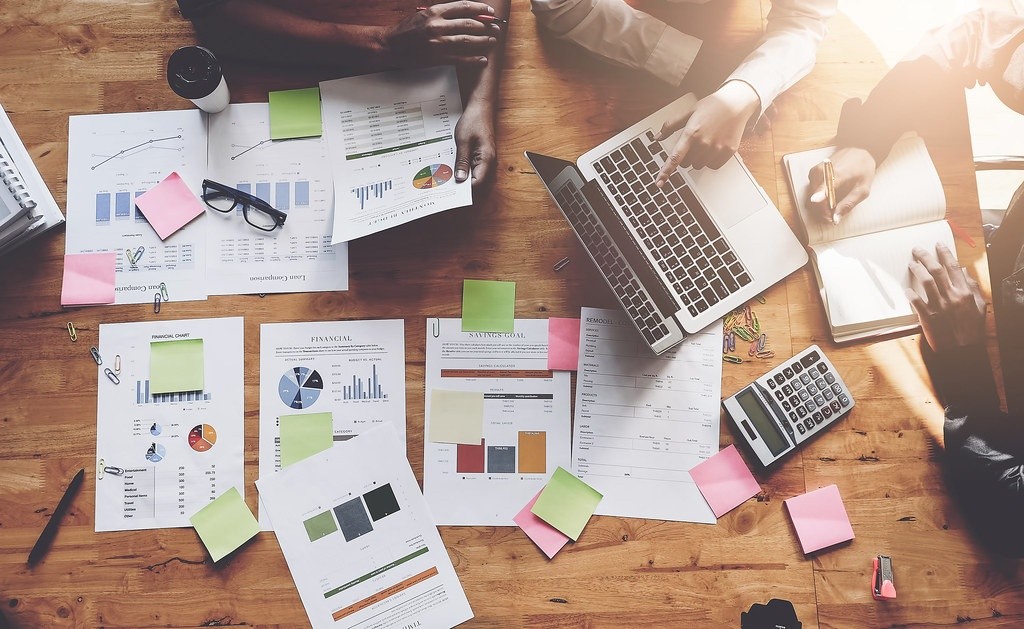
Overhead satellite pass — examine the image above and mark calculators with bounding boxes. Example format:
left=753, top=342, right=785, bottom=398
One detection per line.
left=720, top=344, right=856, bottom=467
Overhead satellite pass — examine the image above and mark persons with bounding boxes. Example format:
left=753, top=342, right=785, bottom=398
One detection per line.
left=529, top=0, right=838, bottom=187
left=178, top=0, right=510, bottom=187
left=811, top=0, right=1024, bottom=563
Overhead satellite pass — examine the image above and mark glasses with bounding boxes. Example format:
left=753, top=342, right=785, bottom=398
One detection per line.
left=200, top=177, right=287, bottom=232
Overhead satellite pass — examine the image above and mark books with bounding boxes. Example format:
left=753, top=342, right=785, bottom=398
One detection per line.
left=0, top=105, right=66, bottom=257
left=784, top=132, right=961, bottom=347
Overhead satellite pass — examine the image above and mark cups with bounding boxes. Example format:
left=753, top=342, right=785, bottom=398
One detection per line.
left=165, top=45, right=231, bottom=114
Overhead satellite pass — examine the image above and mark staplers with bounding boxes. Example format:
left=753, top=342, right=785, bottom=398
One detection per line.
left=872, top=555, right=897, bottom=601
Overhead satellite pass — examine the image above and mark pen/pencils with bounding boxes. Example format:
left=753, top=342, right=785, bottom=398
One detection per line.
left=416, top=7, right=507, bottom=24
left=27, top=468, right=84, bottom=566
left=823, top=158, right=836, bottom=228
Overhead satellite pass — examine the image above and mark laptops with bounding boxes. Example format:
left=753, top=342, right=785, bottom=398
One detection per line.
left=522, top=93, right=809, bottom=355
